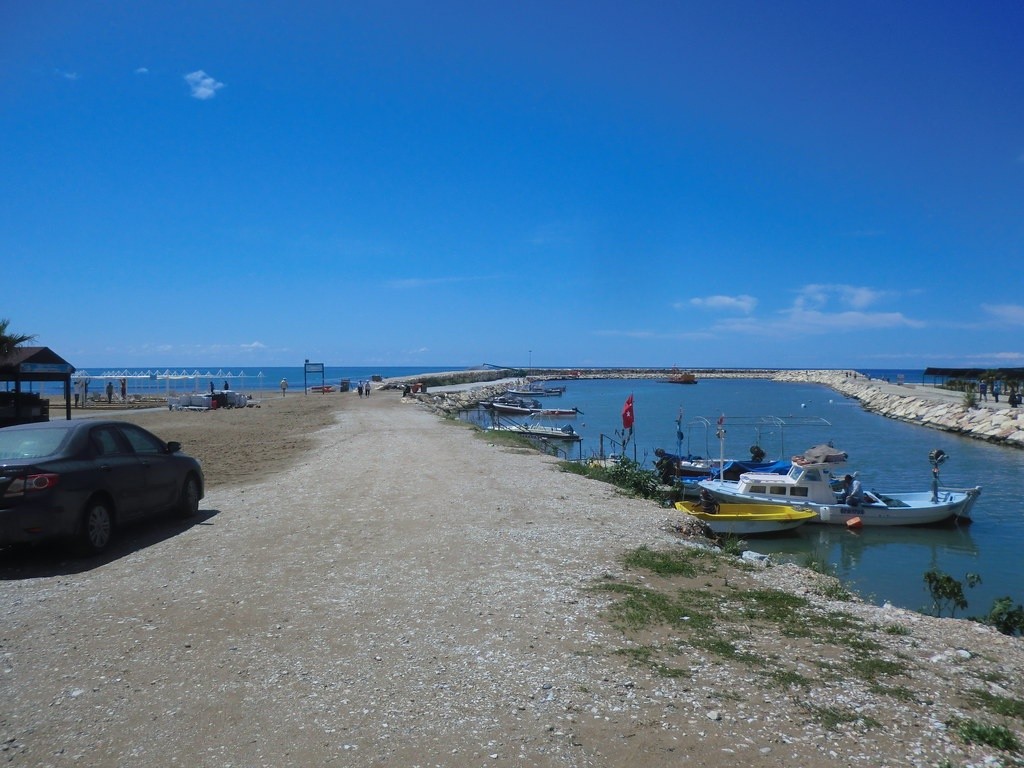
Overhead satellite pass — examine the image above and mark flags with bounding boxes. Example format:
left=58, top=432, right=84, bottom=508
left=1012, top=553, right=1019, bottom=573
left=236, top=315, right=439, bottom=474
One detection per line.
left=622, top=395, right=634, bottom=429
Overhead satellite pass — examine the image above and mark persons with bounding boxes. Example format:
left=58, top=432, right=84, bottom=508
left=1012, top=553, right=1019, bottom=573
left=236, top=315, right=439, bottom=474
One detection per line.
left=358, top=381, right=364, bottom=398
left=1008, top=387, right=1017, bottom=408
left=120, top=378, right=126, bottom=400
left=841, top=475, right=863, bottom=507
left=421, top=384, right=427, bottom=392
left=280, top=378, right=288, bottom=397
left=403, top=384, right=410, bottom=397
left=106, top=382, right=113, bottom=403
left=992, top=385, right=999, bottom=403
left=365, top=380, right=370, bottom=399
left=85, top=378, right=90, bottom=402
left=691, top=489, right=720, bottom=515
left=656, top=458, right=673, bottom=481
left=412, top=385, right=418, bottom=393
left=210, top=382, right=214, bottom=392
left=74, top=381, right=82, bottom=408
left=978, top=380, right=987, bottom=403
left=224, top=381, right=228, bottom=390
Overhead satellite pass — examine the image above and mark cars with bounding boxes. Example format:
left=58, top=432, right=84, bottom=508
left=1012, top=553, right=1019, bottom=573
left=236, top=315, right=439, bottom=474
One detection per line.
left=0, top=417, right=205, bottom=553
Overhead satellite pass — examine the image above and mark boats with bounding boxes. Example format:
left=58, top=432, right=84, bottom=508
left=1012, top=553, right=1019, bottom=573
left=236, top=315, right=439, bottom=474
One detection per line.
left=677, top=494, right=816, bottom=532
left=508, top=384, right=563, bottom=397
left=670, top=461, right=821, bottom=493
left=492, top=401, right=576, bottom=415
left=702, top=469, right=970, bottom=526
left=656, top=450, right=732, bottom=476
left=488, top=420, right=579, bottom=441
left=480, top=396, right=541, bottom=409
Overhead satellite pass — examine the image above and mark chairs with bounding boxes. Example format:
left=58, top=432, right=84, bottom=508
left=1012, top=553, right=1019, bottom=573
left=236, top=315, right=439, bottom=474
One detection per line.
left=92, top=391, right=146, bottom=403
